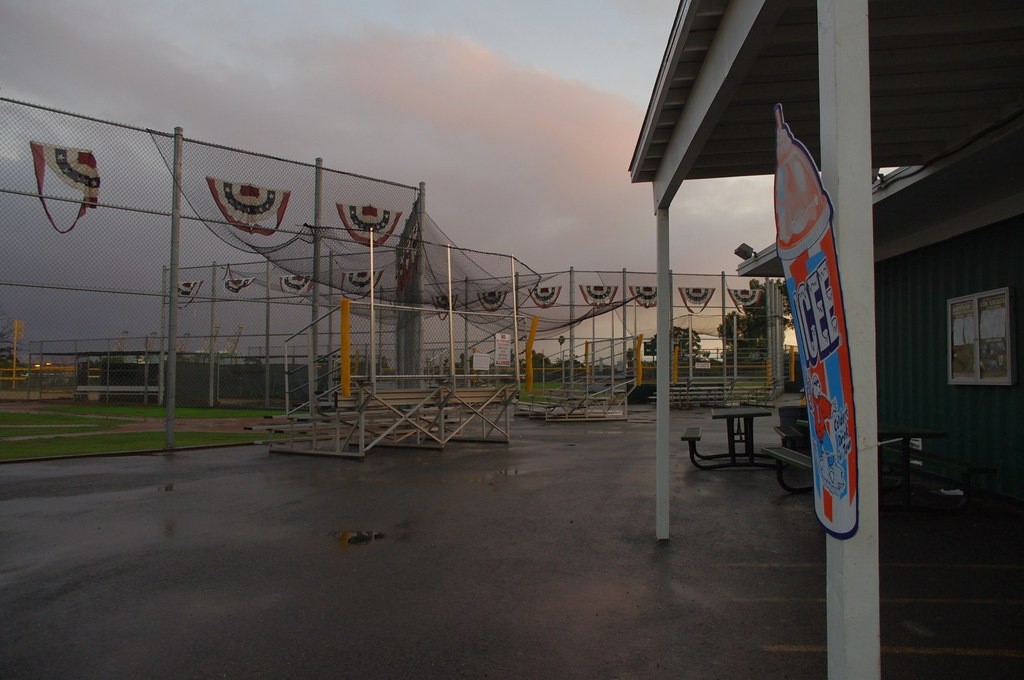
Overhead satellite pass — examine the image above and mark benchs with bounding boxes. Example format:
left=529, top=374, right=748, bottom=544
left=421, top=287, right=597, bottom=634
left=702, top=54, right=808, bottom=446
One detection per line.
left=246, top=385, right=511, bottom=458
left=681, top=427, right=707, bottom=471
left=648, top=376, right=774, bottom=412
left=512, top=379, right=631, bottom=424
left=74, top=385, right=165, bottom=403
left=774, top=425, right=804, bottom=448
left=884, top=446, right=998, bottom=507
left=760, top=445, right=817, bottom=491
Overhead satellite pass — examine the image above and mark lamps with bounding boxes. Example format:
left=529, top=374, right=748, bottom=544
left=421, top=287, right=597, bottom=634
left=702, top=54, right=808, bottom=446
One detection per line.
left=734, top=243, right=757, bottom=260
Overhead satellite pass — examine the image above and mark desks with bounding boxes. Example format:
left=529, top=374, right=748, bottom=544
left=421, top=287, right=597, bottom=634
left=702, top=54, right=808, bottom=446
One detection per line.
left=795, top=418, right=949, bottom=511
left=710, top=407, right=805, bottom=471
